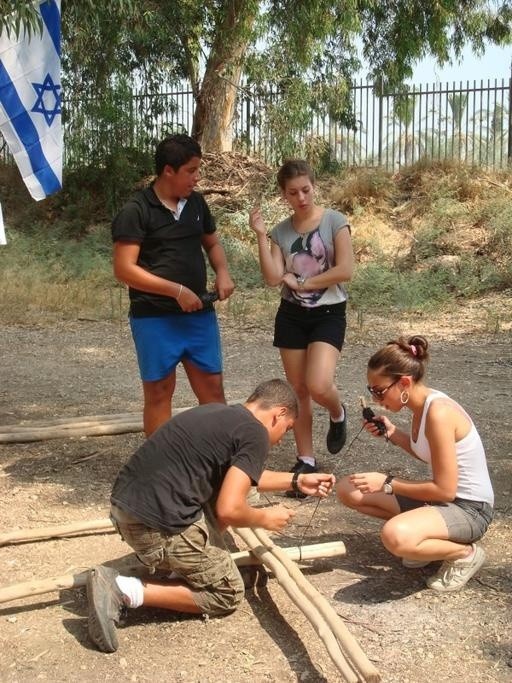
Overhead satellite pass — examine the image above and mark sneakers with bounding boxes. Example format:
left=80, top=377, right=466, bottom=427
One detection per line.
left=287, top=454, right=317, bottom=499
left=397, top=558, right=436, bottom=570
left=324, top=401, right=349, bottom=454
left=425, top=540, right=488, bottom=594
left=84, top=563, right=134, bottom=652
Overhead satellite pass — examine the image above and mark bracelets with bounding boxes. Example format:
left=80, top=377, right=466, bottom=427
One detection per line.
left=175, top=282, right=183, bottom=299
left=297, top=276, right=306, bottom=291
left=292, top=472, right=300, bottom=491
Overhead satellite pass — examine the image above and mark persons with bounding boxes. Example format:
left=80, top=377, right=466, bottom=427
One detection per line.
left=84, top=373, right=340, bottom=654
left=111, top=129, right=227, bottom=434
left=244, top=156, right=355, bottom=493
left=333, top=337, right=496, bottom=594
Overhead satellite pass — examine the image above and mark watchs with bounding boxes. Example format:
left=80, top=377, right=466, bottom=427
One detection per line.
left=383, top=474, right=395, bottom=495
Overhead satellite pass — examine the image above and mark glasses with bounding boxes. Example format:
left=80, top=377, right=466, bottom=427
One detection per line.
left=366, top=373, right=414, bottom=402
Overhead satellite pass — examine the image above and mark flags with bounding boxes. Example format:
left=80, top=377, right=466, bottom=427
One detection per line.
left=1, top=0, right=68, bottom=201
